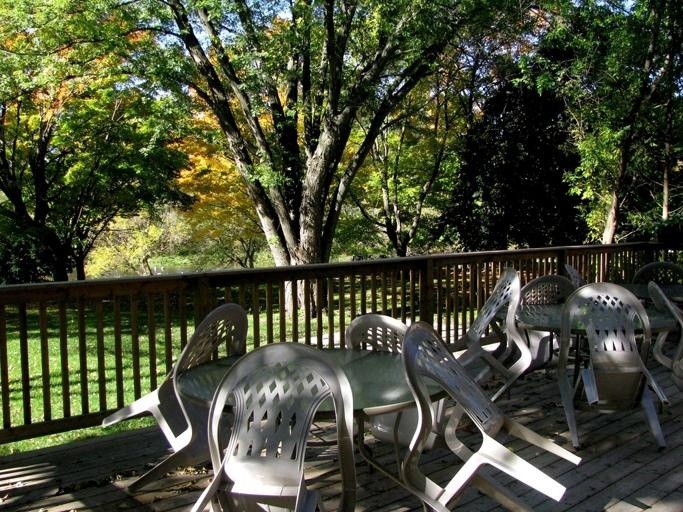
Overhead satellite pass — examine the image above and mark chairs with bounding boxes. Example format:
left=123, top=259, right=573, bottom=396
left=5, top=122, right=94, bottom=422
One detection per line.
left=648, top=281, right=683, bottom=391
left=557, top=280, right=668, bottom=456
left=347, top=313, right=447, bottom=456
left=449, top=268, right=533, bottom=406
left=511, top=273, right=576, bottom=363
left=188, top=341, right=359, bottom=512
left=564, top=264, right=588, bottom=286
left=631, top=263, right=682, bottom=284
left=101, top=303, right=248, bottom=494
left=392, top=317, right=582, bottom=512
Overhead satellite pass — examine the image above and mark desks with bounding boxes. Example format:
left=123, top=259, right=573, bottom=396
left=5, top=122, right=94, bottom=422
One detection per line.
left=613, top=280, right=681, bottom=304
left=177, top=343, right=452, bottom=512
left=494, top=303, right=676, bottom=403
